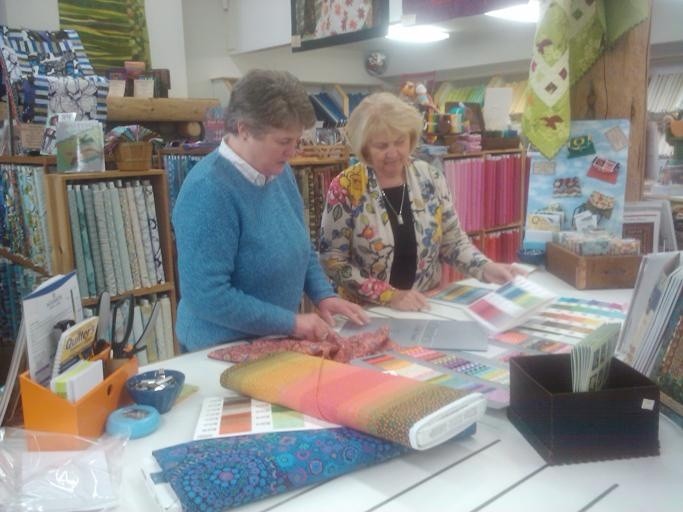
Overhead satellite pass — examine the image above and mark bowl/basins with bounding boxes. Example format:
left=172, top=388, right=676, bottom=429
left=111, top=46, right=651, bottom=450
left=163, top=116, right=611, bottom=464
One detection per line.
left=126, top=369, right=185, bottom=415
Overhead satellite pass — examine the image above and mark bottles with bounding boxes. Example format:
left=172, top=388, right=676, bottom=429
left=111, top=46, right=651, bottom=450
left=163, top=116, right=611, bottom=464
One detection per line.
left=450, top=108, right=464, bottom=135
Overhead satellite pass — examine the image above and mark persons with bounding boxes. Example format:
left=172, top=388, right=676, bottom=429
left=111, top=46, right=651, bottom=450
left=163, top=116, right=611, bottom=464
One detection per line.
left=316, top=89, right=530, bottom=312
left=170, top=69, right=371, bottom=356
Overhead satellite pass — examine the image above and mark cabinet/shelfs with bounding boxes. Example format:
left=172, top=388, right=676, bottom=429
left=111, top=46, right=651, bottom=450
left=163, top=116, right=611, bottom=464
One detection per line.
left=162, top=150, right=525, bottom=314
left=0, top=153, right=182, bottom=385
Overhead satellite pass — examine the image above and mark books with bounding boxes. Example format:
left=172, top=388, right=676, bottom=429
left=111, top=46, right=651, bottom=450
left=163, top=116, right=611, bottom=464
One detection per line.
left=0, top=268, right=113, bottom=428
left=550, top=195, right=682, bottom=258
left=566, top=251, right=683, bottom=428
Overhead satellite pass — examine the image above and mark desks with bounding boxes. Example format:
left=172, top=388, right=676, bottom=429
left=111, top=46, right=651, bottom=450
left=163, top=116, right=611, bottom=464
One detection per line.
left=2, top=265, right=683, bottom=512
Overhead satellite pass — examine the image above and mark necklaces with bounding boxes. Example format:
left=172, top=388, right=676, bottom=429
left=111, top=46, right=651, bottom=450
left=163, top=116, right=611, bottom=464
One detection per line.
left=374, top=171, right=407, bottom=225
